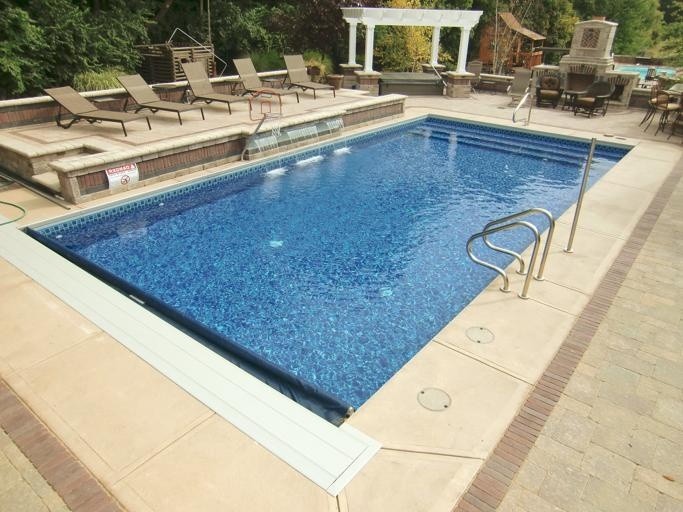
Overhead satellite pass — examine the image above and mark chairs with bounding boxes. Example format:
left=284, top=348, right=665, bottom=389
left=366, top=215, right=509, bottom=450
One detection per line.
left=535, top=68, right=683, bottom=140
left=116, top=73, right=207, bottom=125
left=232, top=58, right=299, bottom=106
left=181, top=60, right=252, bottom=115
left=43, top=85, right=151, bottom=136
left=282, top=54, right=335, bottom=99
